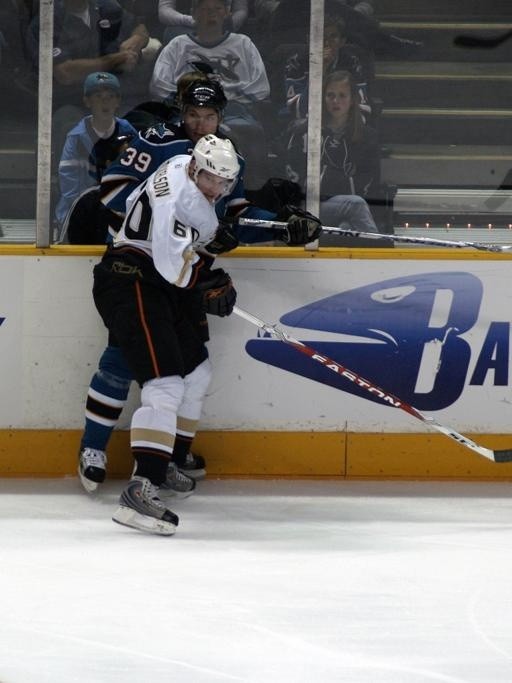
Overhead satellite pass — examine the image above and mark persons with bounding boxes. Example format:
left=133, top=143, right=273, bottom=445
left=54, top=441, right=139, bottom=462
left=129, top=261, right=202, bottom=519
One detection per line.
left=76, top=77, right=320, bottom=492
left=26, top=0, right=423, bottom=247
left=92, top=134, right=252, bottom=537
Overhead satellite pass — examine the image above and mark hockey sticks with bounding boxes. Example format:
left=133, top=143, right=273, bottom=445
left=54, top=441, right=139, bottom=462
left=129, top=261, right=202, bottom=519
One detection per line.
left=231, top=303, right=510, bottom=462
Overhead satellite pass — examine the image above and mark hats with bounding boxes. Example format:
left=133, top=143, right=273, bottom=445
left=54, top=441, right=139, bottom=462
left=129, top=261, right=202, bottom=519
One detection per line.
left=83, top=72, right=121, bottom=96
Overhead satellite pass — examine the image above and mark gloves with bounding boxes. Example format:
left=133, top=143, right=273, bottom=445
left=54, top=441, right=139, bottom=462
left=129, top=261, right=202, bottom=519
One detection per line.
left=204, top=223, right=239, bottom=255
left=281, top=205, right=322, bottom=246
left=196, top=268, right=237, bottom=318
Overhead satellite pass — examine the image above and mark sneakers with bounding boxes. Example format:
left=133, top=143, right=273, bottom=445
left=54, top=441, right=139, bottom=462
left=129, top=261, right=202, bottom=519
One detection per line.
left=80, top=448, right=205, bottom=525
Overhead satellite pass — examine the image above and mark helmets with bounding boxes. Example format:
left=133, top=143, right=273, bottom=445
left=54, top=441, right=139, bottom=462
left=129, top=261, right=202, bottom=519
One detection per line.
left=179, top=79, right=227, bottom=123
left=187, top=133, right=240, bottom=207
